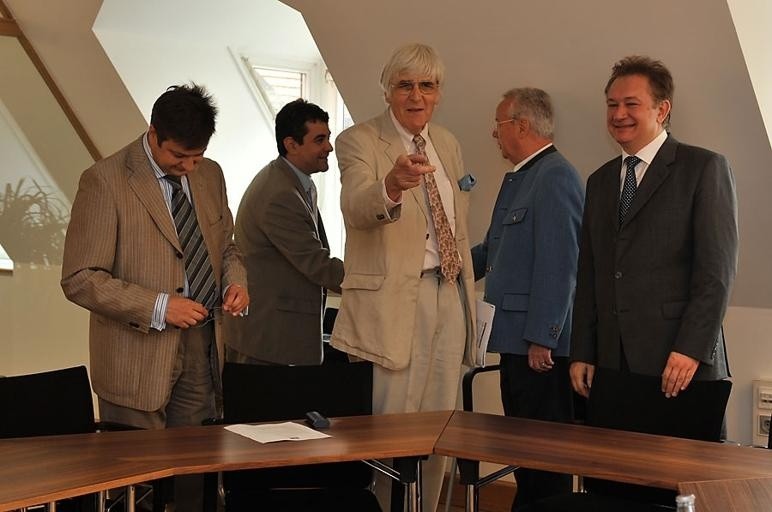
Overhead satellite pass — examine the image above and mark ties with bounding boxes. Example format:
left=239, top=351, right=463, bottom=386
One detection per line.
left=619, top=154, right=642, bottom=224
left=165, top=176, right=221, bottom=312
left=412, top=131, right=464, bottom=286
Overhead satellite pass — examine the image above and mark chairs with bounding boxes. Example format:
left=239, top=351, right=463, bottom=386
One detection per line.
left=519, top=366, right=731, bottom=511
left=2, top=365, right=163, bottom=512
left=201, top=361, right=375, bottom=512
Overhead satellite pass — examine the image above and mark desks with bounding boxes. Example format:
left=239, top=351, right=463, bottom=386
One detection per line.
left=2, top=440, right=179, bottom=512
left=1, top=405, right=455, bottom=512
left=434, top=410, right=772, bottom=512
left=674, top=478, right=772, bottom=512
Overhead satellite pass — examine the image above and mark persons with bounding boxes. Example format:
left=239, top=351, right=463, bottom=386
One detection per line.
left=327, top=40, right=480, bottom=512
left=469, top=85, right=586, bottom=512
left=59, top=81, right=253, bottom=512
left=564, top=54, right=743, bottom=512
left=220, top=96, right=344, bottom=512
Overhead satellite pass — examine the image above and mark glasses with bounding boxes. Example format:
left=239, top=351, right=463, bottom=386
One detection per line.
left=389, top=79, right=439, bottom=95
left=491, top=116, right=520, bottom=129
